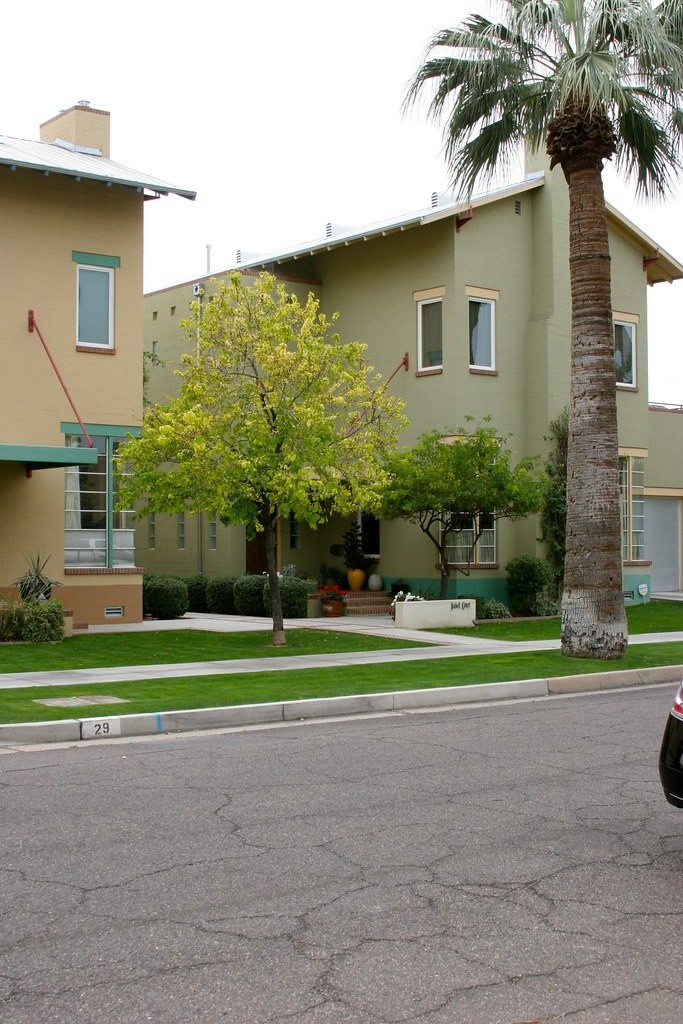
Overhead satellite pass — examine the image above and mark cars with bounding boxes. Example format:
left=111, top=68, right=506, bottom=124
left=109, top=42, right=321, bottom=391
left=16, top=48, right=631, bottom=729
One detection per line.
left=658, top=678, right=683, bottom=808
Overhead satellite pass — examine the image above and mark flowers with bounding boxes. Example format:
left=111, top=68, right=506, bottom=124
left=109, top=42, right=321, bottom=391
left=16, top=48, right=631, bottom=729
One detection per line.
left=318, top=584, right=346, bottom=604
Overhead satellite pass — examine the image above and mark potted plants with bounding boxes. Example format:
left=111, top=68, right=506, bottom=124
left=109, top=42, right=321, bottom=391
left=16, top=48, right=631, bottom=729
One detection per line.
left=341, top=521, right=368, bottom=590
left=7, top=547, right=64, bottom=604
left=391, top=577, right=408, bottom=595
left=319, top=561, right=340, bottom=585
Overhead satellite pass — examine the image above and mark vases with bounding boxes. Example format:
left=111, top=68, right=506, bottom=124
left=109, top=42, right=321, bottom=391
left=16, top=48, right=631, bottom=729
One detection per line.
left=319, top=600, right=343, bottom=616
